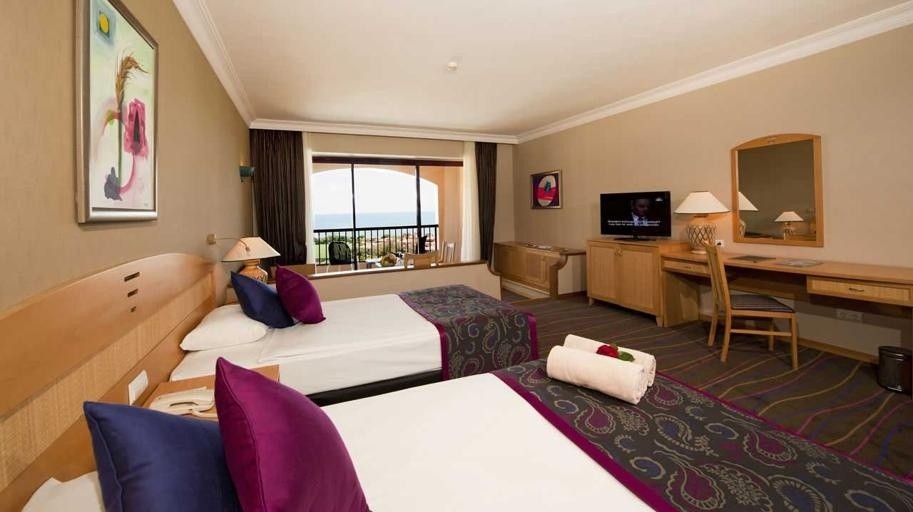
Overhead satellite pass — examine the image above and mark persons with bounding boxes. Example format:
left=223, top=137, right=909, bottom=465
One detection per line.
left=621, top=196, right=654, bottom=228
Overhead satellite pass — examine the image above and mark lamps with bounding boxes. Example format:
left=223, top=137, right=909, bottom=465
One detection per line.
left=673, top=190, right=733, bottom=255
left=219, top=233, right=282, bottom=287
left=206, top=232, right=251, bottom=253
left=774, top=209, right=805, bottom=240
left=238, top=163, right=257, bottom=185
left=735, top=187, right=760, bottom=240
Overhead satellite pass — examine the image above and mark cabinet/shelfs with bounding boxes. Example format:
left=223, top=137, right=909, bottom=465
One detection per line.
left=583, top=233, right=701, bottom=330
left=491, top=237, right=582, bottom=301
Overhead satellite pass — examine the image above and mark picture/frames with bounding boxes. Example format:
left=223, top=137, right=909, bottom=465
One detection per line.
left=69, top=1, right=163, bottom=227
left=527, top=169, right=562, bottom=211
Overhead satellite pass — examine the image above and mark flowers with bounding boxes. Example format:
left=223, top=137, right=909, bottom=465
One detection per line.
left=93, top=44, right=152, bottom=203
left=593, top=340, right=636, bottom=363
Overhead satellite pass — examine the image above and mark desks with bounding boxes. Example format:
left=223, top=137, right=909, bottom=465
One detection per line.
left=658, top=245, right=912, bottom=322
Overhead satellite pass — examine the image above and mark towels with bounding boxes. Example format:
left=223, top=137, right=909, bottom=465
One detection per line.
left=544, top=330, right=649, bottom=408
left=562, top=331, right=658, bottom=389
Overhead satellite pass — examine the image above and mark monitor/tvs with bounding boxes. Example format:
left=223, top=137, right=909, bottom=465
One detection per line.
left=599, top=190, right=672, bottom=241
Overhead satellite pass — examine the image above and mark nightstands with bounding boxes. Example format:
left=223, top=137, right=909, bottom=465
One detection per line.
left=136, top=364, right=283, bottom=434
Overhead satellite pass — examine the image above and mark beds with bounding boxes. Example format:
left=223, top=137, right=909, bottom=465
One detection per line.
left=158, top=280, right=542, bottom=413
left=0, top=335, right=911, bottom=512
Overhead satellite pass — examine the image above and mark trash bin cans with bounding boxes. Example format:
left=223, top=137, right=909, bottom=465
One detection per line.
left=877, top=345, right=913, bottom=394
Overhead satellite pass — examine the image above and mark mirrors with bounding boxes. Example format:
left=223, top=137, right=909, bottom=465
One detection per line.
left=728, top=131, right=826, bottom=250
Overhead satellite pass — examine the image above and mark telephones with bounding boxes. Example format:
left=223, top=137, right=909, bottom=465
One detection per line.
left=148, top=388, right=215, bottom=415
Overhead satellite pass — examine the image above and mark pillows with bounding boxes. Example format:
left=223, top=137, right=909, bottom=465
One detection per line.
left=173, top=301, right=271, bottom=352
left=20, top=466, right=107, bottom=511
left=77, top=397, right=244, bottom=511
left=209, top=353, right=376, bottom=512
left=227, top=270, right=296, bottom=330
left=270, top=263, right=327, bottom=325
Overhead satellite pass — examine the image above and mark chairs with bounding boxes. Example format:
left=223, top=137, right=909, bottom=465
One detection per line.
left=699, top=240, right=801, bottom=375
left=267, top=236, right=457, bottom=281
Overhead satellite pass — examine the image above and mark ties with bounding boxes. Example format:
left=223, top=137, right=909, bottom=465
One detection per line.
left=638, top=217, right=644, bottom=226
